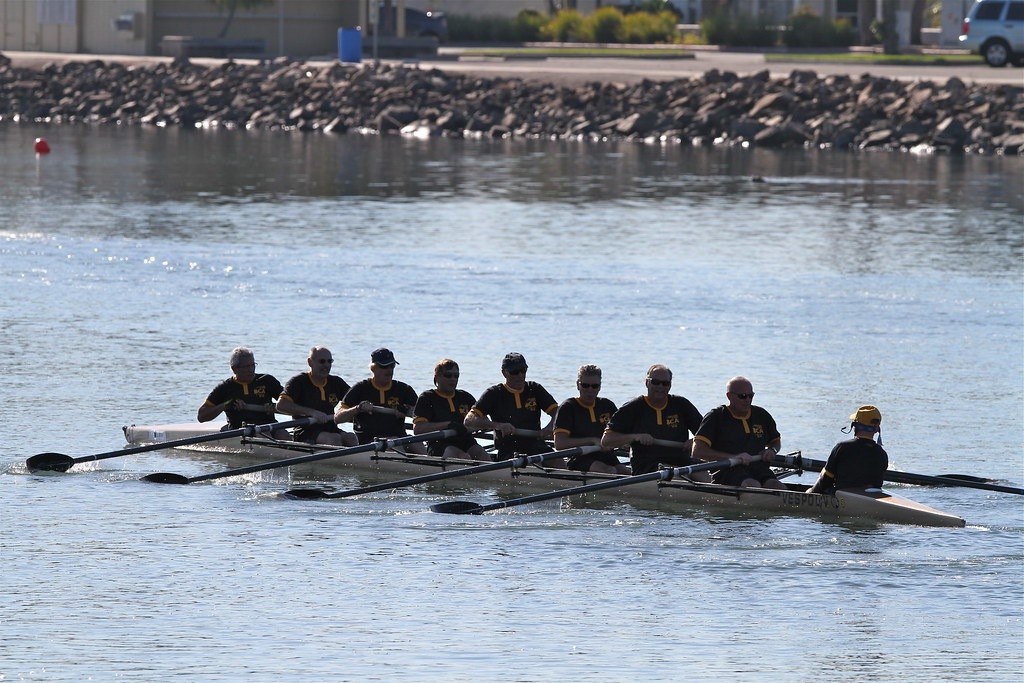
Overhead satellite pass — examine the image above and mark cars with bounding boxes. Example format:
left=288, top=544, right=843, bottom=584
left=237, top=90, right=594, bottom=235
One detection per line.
left=379, top=6, right=449, bottom=51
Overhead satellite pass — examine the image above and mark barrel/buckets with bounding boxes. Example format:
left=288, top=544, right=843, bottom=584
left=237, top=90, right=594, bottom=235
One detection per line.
left=338, top=27, right=362, bottom=62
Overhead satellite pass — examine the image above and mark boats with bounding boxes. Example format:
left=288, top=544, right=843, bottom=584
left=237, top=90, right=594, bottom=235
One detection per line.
left=122, top=423, right=966, bottom=527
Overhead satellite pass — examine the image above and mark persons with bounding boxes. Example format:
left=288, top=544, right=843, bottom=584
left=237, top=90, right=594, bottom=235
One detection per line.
left=601, top=363, right=711, bottom=483
left=553, top=364, right=631, bottom=475
left=463, top=352, right=567, bottom=469
left=198, top=346, right=292, bottom=443
left=276, top=345, right=359, bottom=447
left=334, top=347, right=427, bottom=454
left=804, top=405, right=888, bottom=495
left=413, top=358, right=493, bottom=461
left=691, top=376, right=787, bottom=490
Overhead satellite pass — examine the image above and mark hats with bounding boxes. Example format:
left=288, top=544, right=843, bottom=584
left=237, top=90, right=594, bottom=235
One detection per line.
left=850, top=406, right=881, bottom=426
left=502, top=352, right=528, bottom=370
left=371, top=348, right=400, bottom=366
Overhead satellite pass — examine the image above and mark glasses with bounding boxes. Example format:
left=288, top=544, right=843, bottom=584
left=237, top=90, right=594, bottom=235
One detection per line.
left=508, top=368, right=527, bottom=375
left=579, top=382, right=600, bottom=389
left=647, top=378, right=670, bottom=387
left=236, top=363, right=258, bottom=369
left=377, top=363, right=395, bottom=369
left=443, top=373, right=459, bottom=379
left=730, top=391, right=755, bottom=399
left=319, top=358, right=334, bottom=364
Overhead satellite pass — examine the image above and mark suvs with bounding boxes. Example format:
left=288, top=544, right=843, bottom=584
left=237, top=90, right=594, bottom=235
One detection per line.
left=959, top=0, right=1024, bottom=68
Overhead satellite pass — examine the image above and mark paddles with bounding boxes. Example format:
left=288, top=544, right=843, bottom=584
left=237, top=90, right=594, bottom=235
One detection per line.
left=764, top=454, right=1024, bottom=495
left=429, top=454, right=763, bottom=516
left=26, top=413, right=334, bottom=475
left=281, top=445, right=602, bottom=500
left=138, top=429, right=460, bottom=484
